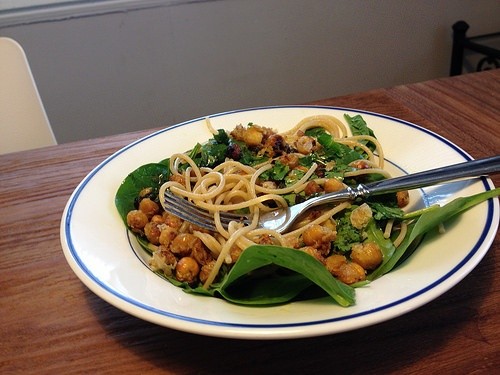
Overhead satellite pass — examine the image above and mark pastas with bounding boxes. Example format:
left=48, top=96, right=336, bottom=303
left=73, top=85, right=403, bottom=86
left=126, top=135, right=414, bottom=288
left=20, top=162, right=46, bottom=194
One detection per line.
left=158, top=116, right=445, bottom=289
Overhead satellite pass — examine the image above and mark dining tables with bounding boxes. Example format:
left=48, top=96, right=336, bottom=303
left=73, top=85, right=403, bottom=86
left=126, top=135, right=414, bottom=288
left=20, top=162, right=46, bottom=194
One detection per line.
left=0, top=69, right=500, bottom=374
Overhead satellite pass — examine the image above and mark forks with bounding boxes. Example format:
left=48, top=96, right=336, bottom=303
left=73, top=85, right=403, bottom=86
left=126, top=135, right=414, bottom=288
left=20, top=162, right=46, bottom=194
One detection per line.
left=163, top=156, right=500, bottom=245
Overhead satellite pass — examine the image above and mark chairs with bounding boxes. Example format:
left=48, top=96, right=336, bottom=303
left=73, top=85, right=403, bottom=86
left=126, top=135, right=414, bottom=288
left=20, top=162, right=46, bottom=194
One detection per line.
left=0, top=37, right=58, bottom=158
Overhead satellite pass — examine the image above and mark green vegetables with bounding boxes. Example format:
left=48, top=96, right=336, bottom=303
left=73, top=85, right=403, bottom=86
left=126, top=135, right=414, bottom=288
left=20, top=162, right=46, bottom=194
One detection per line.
left=114, top=129, right=252, bottom=253
left=364, top=187, right=500, bottom=282
left=163, top=244, right=356, bottom=308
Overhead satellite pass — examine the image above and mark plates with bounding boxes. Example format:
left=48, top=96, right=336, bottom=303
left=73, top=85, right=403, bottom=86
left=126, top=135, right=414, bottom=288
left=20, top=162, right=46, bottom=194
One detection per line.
left=59, top=106, right=498, bottom=341
